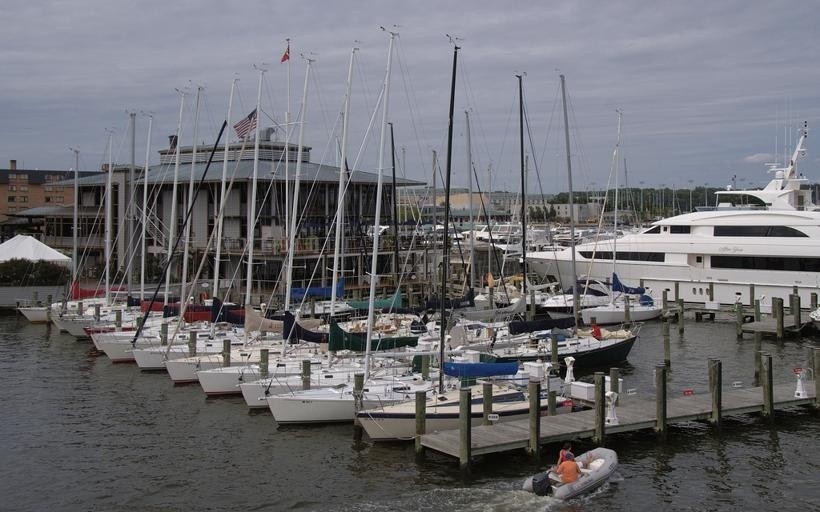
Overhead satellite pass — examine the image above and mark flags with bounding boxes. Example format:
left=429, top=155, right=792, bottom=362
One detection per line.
left=280, top=45, right=290, bottom=64
left=231, top=105, right=257, bottom=140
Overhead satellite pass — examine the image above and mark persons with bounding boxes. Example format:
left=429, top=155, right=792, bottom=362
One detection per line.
left=556, top=442, right=574, bottom=469
left=553, top=452, right=581, bottom=485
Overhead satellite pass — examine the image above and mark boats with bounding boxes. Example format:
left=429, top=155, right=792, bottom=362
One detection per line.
left=524, top=117, right=820, bottom=323
left=522, top=447, right=621, bottom=502
